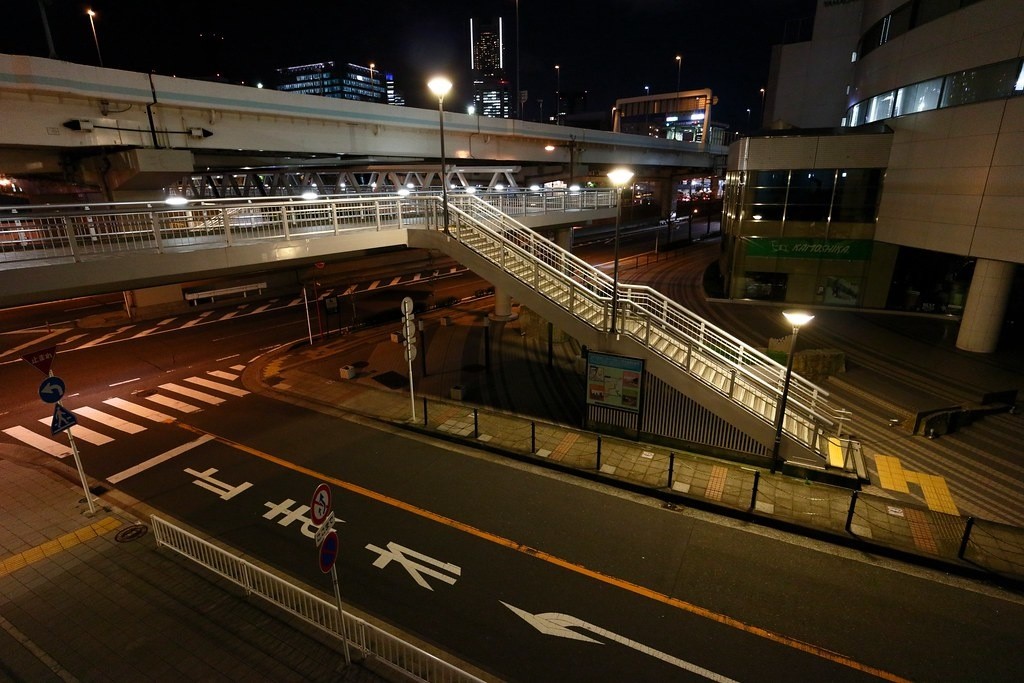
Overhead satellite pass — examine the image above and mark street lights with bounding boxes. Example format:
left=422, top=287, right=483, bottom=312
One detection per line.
left=606, top=166, right=636, bottom=334
left=370, top=63, right=376, bottom=102
left=87, top=9, right=105, bottom=67
left=674, top=56, right=682, bottom=140
left=760, top=88, right=765, bottom=130
left=428, top=75, right=453, bottom=234
left=555, top=65, right=560, bottom=125
left=769, top=307, right=818, bottom=475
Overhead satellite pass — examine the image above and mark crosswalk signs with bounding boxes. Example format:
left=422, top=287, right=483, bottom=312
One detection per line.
left=50, top=403, right=77, bottom=436
